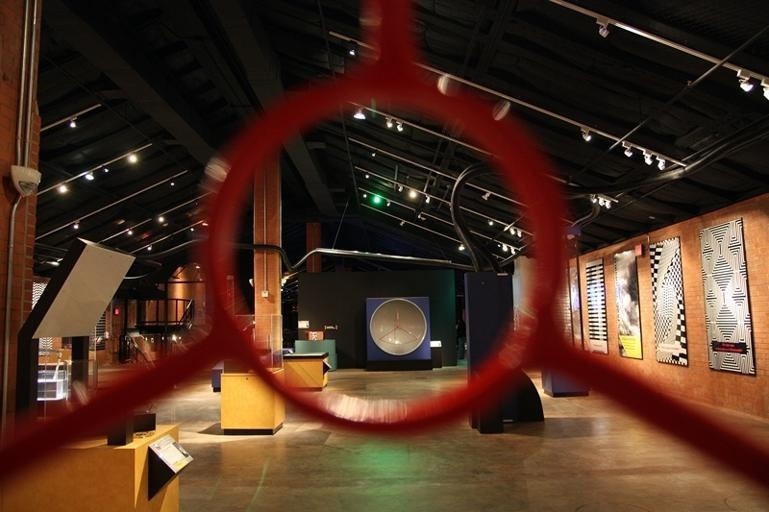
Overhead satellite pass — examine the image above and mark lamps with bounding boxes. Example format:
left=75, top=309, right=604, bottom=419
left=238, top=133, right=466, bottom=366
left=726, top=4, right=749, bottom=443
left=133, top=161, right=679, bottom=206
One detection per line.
left=583, top=132, right=591, bottom=141
left=624, top=149, right=633, bottom=157
left=599, top=25, right=610, bottom=39
left=354, top=110, right=367, bottom=121
left=644, top=154, right=652, bottom=166
left=396, top=124, right=404, bottom=133
left=386, top=120, right=393, bottom=128
left=656, top=157, right=666, bottom=172
left=737, top=71, right=753, bottom=93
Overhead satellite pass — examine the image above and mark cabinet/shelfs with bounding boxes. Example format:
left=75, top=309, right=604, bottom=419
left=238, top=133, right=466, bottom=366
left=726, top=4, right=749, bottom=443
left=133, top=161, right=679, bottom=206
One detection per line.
left=219, top=368, right=283, bottom=437
left=541, top=370, right=590, bottom=398
left=282, top=351, right=330, bottom=391
left=0, top=424, right=179, bottom=511
